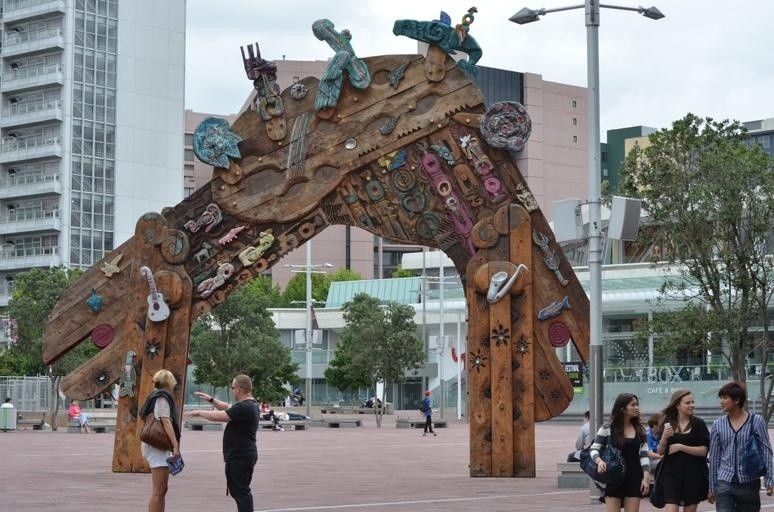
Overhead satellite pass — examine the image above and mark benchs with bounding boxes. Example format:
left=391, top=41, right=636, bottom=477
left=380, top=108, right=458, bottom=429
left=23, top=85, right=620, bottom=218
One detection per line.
left=258, top=420, right=311, bottom=431
left=184, top=418, right=223, bottom=431
left=327, top=420, right=363, bottom=429
left=395, top=419, right=449, bottom=429
left=555, top=461, right=590, bottom=489
left=17, top=419, right=45, bottom=429
left=67, top=422, right=117, bottom=433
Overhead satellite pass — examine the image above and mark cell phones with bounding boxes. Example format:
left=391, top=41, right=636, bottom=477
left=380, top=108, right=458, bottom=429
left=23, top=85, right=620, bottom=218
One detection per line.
left=664, top=422, right=674, bottom=437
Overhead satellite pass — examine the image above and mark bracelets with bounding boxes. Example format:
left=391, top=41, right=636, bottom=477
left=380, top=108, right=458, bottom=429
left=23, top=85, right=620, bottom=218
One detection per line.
left=208, top=397, right=214, bottom=402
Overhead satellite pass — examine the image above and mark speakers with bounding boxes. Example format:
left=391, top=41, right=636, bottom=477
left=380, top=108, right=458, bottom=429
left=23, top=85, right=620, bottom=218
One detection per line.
left=607, top=195, right=641, bottom=242
left=551, top=198, right=583, bottom=243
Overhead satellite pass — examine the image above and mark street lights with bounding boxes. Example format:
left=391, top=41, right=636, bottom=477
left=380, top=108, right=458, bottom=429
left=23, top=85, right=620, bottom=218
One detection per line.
left=406, top=244, right=466, bottom=422
left=283, top=238, right=333, bottom=423
left=508, top=0, right=665, bottom=490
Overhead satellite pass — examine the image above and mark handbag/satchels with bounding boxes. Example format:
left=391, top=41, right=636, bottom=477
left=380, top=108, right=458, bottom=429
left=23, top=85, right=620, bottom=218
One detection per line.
left=419, top=400, right=427, bottom=412
left=139, top=413, right=176, bottom=452
left=580, top=440, right=627, bottom=485
left=741, top=436, right=767, bottom=477
left=649, top=483, right=665, bottom=509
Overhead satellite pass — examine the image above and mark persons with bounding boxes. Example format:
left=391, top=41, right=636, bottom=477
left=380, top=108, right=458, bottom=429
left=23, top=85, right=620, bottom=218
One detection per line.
left=358, top=395, right=381, bottom=414
left=184, top=374, right=260, bottom=512
left=422, top=391, right=438, bottom=436
left=69, top=399, right=91, bottom=433
left=5, top=398, right=12, bottom=405
left=260, top=384, right=311, bottom=432
left=138, top=369, right=181, bottom=512
left=567, top=382, right=774, bottom=512
